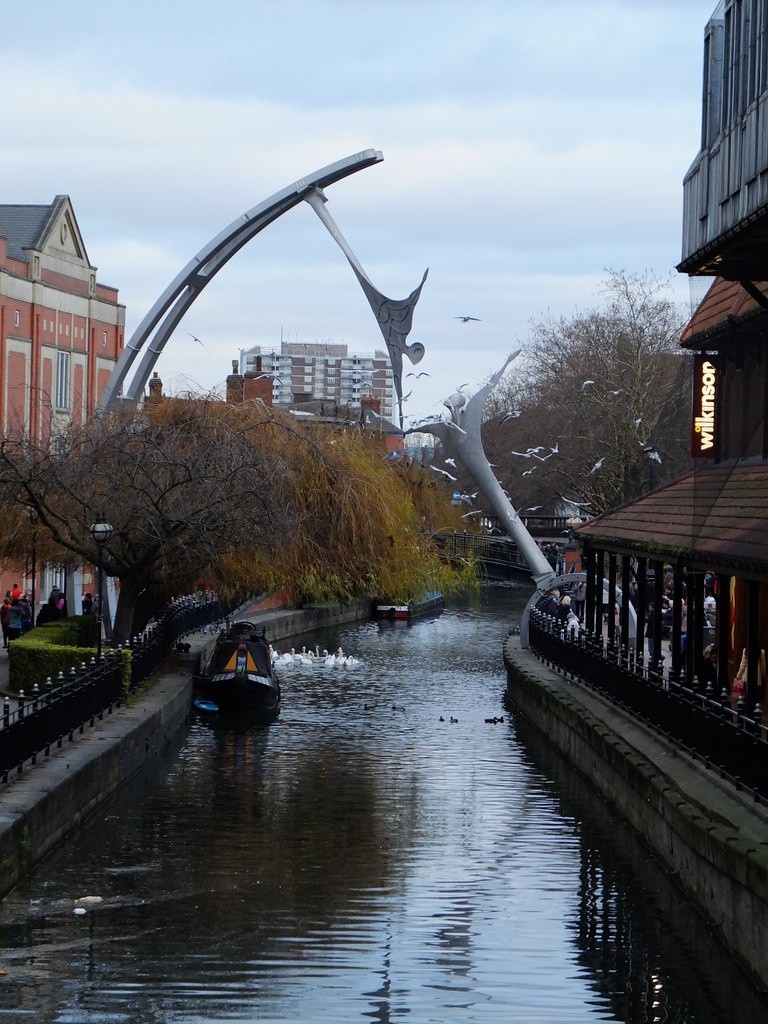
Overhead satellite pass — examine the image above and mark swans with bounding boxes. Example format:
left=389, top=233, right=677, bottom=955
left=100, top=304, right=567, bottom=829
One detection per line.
left=268, top=645, right=359, bottom=667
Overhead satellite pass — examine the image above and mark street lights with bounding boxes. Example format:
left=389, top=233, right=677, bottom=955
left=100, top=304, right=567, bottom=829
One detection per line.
left=25, top=506, right=40, bottom=627
left=88, top=511, right=114, bottom=668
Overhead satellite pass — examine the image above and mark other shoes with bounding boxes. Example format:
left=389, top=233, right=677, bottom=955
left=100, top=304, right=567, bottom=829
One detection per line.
left=3, top=646, right=8, bottom=649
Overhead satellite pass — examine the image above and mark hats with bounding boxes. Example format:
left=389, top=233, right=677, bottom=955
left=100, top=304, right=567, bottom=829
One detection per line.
left=85, top=593, right=92, bottom=600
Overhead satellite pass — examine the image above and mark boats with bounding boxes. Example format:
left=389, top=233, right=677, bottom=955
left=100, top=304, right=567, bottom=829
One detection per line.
left=193, top=620, right=280, bottom=715
left=374, top=591, right=445, bottom=621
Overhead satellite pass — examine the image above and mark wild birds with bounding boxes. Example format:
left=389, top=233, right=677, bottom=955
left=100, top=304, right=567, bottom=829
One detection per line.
left=253, top=373, right=283, bottom=385
left=338, top=372, right=662, bottom=534
left=451, top=315, right=481, bottom=323
left=363, top=704, right=370, bottom=710
left=450, top=717, right=459, bottom=723
left=484, top=716, right=504, bottom=723
left=391, top=706, right=405, bottom=712
left=439, top=715, right=445, bottom=721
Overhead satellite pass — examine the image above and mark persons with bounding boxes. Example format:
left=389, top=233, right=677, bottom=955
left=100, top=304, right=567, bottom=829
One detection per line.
left=0, top=584, right=32, bottom=652
left=196, top=571, right=214, bottom=608
left=83, top=593, right=99, bottom=622
left=549, top=557, right=768, bottom=713
left=155, top=596, right=176, bottom=628
left=36, top=586, right=64, bottom=627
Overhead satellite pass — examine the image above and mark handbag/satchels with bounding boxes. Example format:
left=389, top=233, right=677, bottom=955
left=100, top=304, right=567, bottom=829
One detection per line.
left=731, top=678, right=744, bottom=703
